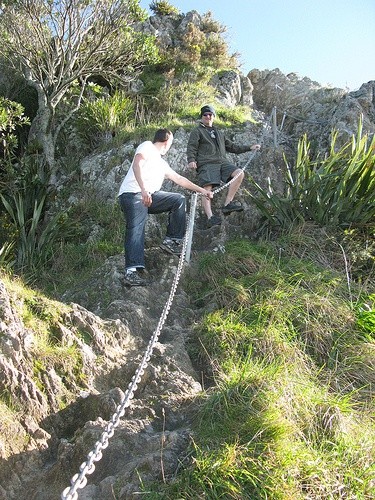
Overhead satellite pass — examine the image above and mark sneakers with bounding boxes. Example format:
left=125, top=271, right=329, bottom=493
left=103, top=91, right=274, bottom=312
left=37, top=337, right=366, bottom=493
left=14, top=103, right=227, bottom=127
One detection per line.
left=223, top=204, right=243, bottom=213
left=124, top=271, right=144, bottom=286
left=207, top=216, right=223, bottom=227
left=160, top=240, right=192, bottom=258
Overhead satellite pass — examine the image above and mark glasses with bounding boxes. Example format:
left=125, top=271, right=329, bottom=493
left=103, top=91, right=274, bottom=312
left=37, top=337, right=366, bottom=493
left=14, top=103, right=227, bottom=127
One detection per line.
left=204, top=113, right=212, bottom=116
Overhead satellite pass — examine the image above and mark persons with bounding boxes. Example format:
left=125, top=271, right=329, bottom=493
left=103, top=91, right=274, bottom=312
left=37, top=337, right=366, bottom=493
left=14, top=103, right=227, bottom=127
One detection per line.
left=117, top=128, right=213, bottom=286
left=187, top=105, right=261, bottom=228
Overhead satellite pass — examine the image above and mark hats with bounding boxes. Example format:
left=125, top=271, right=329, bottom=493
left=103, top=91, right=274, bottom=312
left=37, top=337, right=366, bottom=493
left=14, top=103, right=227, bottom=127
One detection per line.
left=201, top=105, right=215, bottom=117
